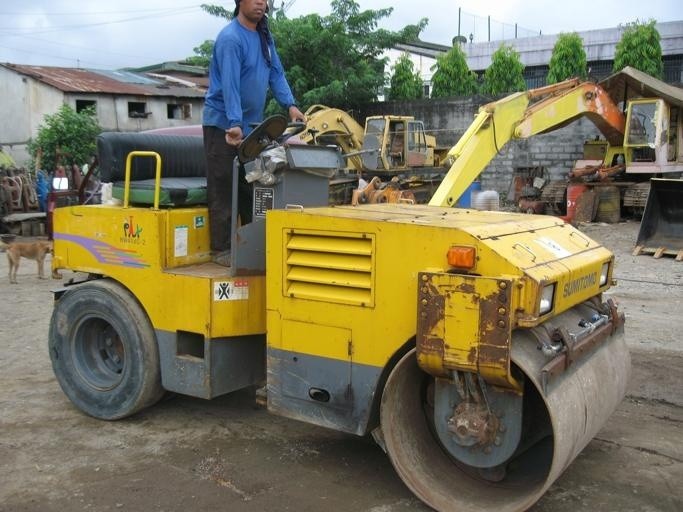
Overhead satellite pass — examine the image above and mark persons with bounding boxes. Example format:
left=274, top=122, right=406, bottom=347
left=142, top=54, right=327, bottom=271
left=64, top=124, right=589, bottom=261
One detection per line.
left=199, top=0, right=307, bottom=267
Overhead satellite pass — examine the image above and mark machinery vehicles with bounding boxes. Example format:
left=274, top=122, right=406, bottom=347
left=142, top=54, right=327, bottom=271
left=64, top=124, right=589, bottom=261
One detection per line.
left=353, top=65, right=683, bottom=226
left=49, top=114, right=632, bottom=512
left=283, top=103, right=451, bottom=205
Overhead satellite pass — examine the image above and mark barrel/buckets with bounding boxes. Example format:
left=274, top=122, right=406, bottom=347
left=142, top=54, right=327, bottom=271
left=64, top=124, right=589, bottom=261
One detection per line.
left=458, top=181, right=481, bottom=207
left=566, top=183, right=589, bottom=221
left=470, top=189, right=500, bottom=211
left=594, top=184, right=620, bottom=224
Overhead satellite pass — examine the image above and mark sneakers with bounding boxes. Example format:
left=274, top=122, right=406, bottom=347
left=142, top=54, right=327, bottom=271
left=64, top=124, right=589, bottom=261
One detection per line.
left=212, top=250, right=231, bottom=267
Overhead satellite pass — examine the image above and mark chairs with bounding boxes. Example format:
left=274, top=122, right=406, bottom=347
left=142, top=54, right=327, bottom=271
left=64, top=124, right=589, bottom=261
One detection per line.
left=388, top=130, right=404, bottom=158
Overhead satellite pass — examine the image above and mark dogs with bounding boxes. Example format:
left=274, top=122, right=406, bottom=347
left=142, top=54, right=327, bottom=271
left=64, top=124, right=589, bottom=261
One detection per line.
left=0, top=238, right=54, bottom=284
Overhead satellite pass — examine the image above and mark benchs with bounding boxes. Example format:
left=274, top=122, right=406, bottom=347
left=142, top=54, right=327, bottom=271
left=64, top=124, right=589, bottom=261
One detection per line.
left=97, top=131, right=209, bottom=206
